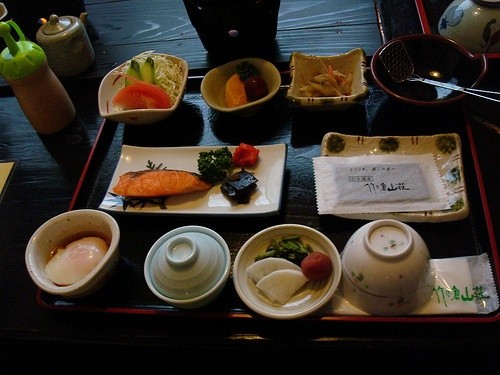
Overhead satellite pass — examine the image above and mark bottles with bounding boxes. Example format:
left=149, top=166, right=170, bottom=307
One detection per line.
left=0, top=20, right=77, bottom=134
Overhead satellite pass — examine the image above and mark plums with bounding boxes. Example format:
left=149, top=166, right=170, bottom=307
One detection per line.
left=245, top=75, right=269, bottom=98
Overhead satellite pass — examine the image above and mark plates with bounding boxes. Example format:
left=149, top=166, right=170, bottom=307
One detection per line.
left=98, top=143, right=288, bottom=216
left=322, top=131, right=469, bottom=223
left=201, top=57, right=281, bottom=117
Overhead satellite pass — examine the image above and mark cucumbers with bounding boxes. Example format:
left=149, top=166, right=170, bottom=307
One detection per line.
left=139, top=56, right=155, bottom=83
left=124, top=60, right=140, bottom=86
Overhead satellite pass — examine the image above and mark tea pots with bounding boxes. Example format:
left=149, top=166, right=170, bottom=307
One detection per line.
left=35, top=12, right=96, bottom=77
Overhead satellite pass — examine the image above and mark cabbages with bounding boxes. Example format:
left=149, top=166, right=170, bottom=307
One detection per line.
left=117, top=52, right=182, bottom=106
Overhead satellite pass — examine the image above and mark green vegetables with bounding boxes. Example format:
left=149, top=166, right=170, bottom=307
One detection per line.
left=196, top=146, right=233, bottom=183
left=108, top=160, right=167, bottom=209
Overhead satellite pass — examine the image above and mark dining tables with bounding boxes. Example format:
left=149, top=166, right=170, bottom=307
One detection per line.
left=0, top=0, right=500, bottom=343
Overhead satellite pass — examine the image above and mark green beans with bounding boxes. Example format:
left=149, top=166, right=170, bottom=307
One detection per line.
left=254, top=235, right=315, bottom=266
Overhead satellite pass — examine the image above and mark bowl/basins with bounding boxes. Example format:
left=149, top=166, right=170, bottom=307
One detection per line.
left=98, top=53, right=188, bottom=125
left=437, top=0, right=500, bottom=54
left=232, top=223, right=343, bottom=320
left=338, top=219, right=436, bottom=317
left=370, top=33, right=487, bottom=114
left=285, top=47, right=368, bottom=109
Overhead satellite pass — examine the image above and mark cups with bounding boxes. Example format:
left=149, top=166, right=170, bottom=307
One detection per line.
left=143, top=225, right=230, bottom=310
left=24, top=209, right=120, bottom=300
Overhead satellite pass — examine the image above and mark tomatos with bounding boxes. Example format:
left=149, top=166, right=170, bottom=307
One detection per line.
left=112, top=75, right=171, bottom=111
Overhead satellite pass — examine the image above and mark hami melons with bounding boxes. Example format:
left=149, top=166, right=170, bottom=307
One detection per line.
left=225, top=73, right=248, bottom=107
